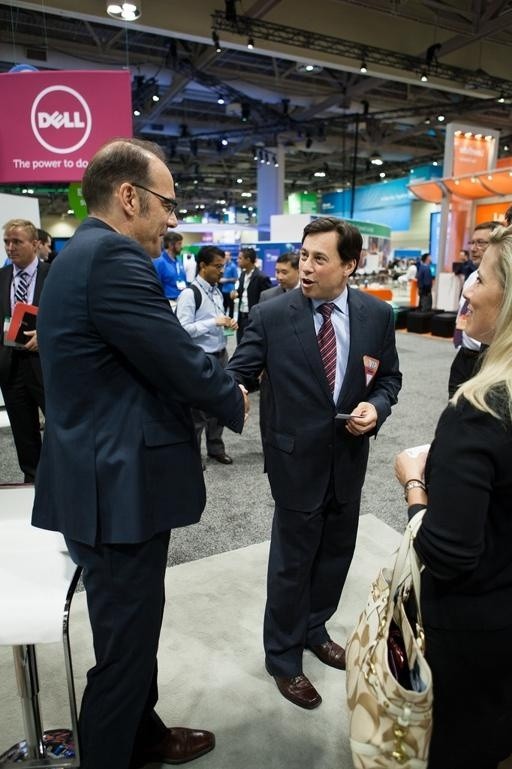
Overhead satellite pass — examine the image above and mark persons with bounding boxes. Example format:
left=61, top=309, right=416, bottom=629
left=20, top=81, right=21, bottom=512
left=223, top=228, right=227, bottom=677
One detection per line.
left=223, top=217, right=404, bottom=710
left=0, top=218, right=52, bottom=484
left=348, top=207, right=512, bottom=399
left=30, top=135, right=250, bottom=768
left=394, top=221, right=512, bottom=768
left=153, top=231, right=301, bottom=469
left=3, top=229, right=58, bottom=431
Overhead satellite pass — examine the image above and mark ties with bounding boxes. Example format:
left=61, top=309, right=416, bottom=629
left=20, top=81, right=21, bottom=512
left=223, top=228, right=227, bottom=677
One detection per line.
left=13, top=272, right=29, bottom=306
left=316, top=303, right=336, bottom=398
left=454, top=300, right=470, bottom=347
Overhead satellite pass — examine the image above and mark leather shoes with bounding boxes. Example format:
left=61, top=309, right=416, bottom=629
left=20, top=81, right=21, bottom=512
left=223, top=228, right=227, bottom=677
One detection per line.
left=165, top=727, right=215, bottom=765
left=306, top=640, right=345, bottom=672
left=264, top=663, right=321, bottom=709
left=208, top=453, right=232, bottom=464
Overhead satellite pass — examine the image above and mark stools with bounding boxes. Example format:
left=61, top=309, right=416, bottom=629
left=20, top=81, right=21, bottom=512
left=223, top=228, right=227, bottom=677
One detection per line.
left=0, top=477, right=91, bottom=769
left=390, top=303, right=418, bottom=331
left=430, top=311, right=459, bottom=339
left=408, top=309, right=444, bottom=336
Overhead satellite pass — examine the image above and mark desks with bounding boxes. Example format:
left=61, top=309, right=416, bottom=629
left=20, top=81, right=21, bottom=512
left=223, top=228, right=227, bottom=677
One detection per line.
left=359, top=289, right=393, bottom=309
left=409, top=277, right=436, bottom=308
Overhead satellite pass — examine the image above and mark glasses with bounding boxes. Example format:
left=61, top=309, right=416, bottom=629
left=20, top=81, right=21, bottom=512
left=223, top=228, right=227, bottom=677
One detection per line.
left=467, top=240, right=488, bottom=248
left=131, top=182, right=178, bottom=215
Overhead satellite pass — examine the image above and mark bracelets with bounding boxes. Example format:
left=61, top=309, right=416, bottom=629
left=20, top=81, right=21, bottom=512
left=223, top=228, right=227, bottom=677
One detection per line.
left=402, top=478, right=426, bottom=503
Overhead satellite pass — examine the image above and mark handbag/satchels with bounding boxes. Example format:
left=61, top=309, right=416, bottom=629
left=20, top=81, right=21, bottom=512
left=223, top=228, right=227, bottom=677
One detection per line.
left=346, top=509, right=434, bottom=768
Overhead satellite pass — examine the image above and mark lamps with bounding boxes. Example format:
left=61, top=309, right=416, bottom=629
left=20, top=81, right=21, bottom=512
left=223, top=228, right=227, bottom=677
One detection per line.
left=420, top=67, right=428, bottom=82
left=105, top=1, right=144, bottom=24
left=130, top=77, right=512, bottom=214
left=247, top=36, right=255, bottom=50
left=305, top=64, right=314, bottom=72
left=359, top=59, right=369, bottom=74
left=212, top=28, right=219, bottom=42
left=214, top=42, right=222, bottom=53
